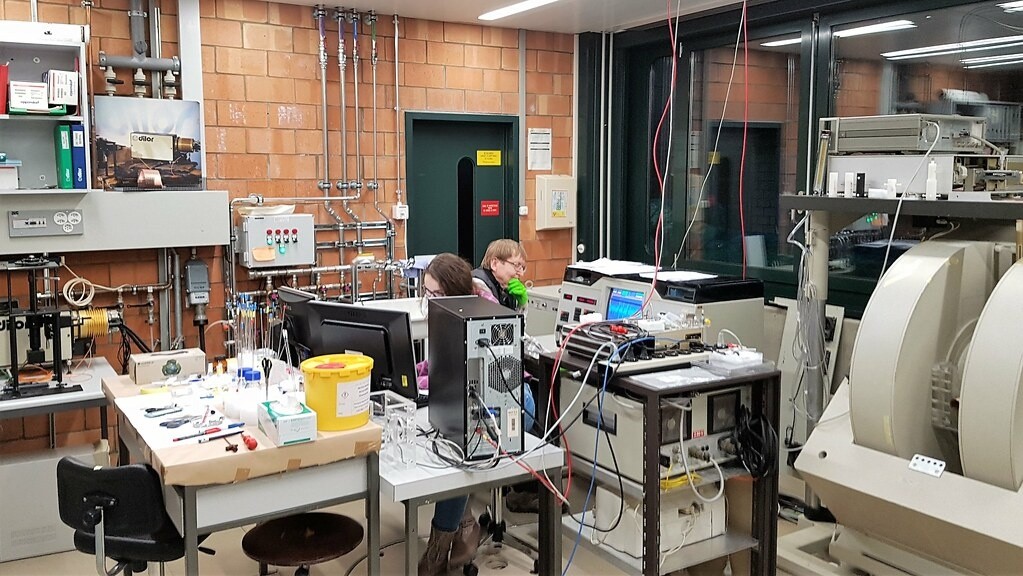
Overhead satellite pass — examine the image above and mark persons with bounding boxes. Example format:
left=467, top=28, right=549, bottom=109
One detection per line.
left=418, top=253, right=534, bottom=576
left=472, top=239, right=569, bottom=514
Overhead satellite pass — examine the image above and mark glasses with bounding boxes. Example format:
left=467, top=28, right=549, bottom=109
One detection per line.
left=495, top=256, right=525, bottom=272
left=422, top=285, right=446, bottom=298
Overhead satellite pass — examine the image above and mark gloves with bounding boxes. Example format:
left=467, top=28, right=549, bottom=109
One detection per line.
left=507, top=277, right=528, bottom=306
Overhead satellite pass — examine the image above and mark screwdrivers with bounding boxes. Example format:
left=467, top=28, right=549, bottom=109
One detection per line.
left=241, top=433, right=258, bottom=450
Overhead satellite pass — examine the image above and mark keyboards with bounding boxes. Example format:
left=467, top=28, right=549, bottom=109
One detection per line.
left=414, top=395, right=429, bottom=410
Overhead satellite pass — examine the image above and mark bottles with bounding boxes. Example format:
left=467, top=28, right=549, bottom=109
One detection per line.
left=235, top=367, right=266, bottom=427
left=213, top=354, right=227, bottom=374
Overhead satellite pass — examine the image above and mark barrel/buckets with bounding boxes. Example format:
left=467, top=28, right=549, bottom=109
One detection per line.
left=300, top=353, right=374, bottom=432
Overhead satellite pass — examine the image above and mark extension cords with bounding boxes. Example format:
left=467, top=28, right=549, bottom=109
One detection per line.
left=597, top=343, right=745, bottom=376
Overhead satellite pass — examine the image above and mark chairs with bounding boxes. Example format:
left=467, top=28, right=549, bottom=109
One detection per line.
left=55, top=454, right=214, bottom=576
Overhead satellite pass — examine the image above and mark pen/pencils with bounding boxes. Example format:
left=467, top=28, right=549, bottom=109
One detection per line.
left=189, top=380, right=201, bottom=382
left=199, top=431, right=244, bottom=444
left=261, top=358, right=272, bottom=401
left=173, top=428, right=221, bottom=443
left=200, top=396, right=214, bottom=399
left=219, top=422, right=245, bottom=430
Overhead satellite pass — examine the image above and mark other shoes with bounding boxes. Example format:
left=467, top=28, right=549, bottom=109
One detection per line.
left=506, top=490, right=570, bottom=514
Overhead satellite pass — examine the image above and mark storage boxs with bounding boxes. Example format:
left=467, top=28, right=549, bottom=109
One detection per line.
left=6, top=80, right=49, bottom=113
left=129, top=348, right=206, bottom=385
left=41, top=69, right=78, bottom=107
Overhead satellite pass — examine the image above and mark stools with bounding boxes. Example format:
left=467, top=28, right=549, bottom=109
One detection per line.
left=241, top=510, right=364, bottom=576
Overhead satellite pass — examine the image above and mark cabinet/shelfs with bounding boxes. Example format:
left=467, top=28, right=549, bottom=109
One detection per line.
left=0, top=20, right=88, bottom=195
left=535, top=351, right=780, bottom=576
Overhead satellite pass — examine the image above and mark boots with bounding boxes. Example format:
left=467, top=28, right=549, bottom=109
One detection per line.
left=449, top=510, right=482, bottom=571
left=418, top=521, right=459, bottom=576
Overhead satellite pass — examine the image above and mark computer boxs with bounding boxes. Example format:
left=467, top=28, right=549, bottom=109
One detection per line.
left=428, top=296, right=526, bottom=464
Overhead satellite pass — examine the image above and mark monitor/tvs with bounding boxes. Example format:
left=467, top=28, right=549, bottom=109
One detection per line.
left=277, top=285, right=419, bottom=401
left=606, top=288, right=644, bottom=321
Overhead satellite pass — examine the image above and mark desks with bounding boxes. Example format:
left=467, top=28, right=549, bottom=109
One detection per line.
left=100, top=355, right=382, bottom=576
left=374, top=402, right=566, bottom=576
